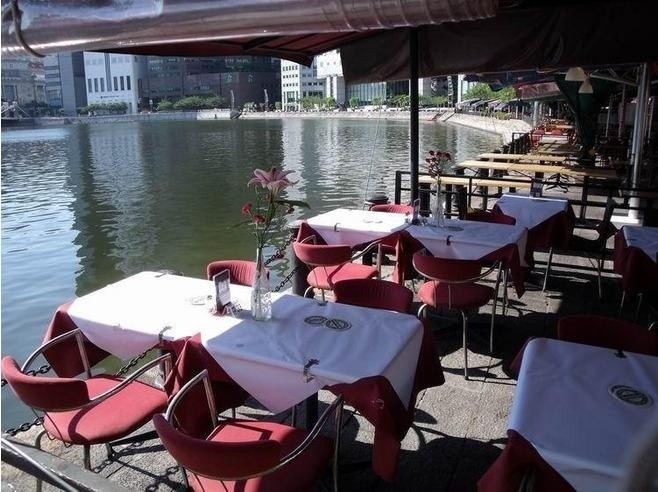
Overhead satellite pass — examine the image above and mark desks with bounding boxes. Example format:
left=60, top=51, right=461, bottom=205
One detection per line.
left=412, top=118, right=654, bottom=235
left=474, top=338, right=656, bottom=492
left=41, top=268, right=444, bottom=482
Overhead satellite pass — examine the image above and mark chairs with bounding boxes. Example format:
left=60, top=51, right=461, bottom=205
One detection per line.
left=1, top=325, right=193, bottom=492
left=152, top=367, right=346, bottom=492
left=288, top=191, right=657, bottom=379
left=206, top=258, right=271, bottom=289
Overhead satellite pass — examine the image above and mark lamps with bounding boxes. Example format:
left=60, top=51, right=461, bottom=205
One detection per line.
left=565, top=65, right=586, bottom=83
left=577, top=72, right=594, bottom=94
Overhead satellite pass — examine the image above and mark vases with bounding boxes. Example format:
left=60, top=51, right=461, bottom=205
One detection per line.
left=246, top=248, right=274, bottom=320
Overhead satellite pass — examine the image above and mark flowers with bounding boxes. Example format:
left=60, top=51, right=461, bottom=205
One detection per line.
left=222, top=163, right=313, bottom=299
